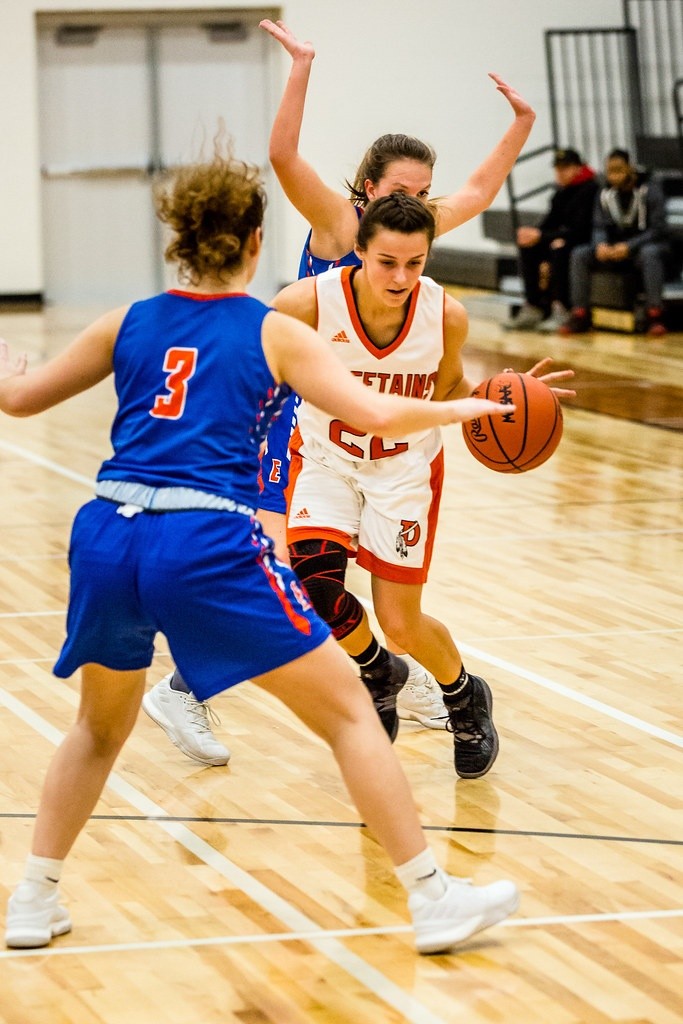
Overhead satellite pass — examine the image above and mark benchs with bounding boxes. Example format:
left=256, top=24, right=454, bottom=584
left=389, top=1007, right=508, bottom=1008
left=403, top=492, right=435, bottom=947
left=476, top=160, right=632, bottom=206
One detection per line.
left=424, top=136, right=683, bottom=331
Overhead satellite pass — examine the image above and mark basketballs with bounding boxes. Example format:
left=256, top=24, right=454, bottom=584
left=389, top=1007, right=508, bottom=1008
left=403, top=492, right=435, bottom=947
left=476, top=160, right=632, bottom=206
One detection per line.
left=463, top=373, right=564, bottom=474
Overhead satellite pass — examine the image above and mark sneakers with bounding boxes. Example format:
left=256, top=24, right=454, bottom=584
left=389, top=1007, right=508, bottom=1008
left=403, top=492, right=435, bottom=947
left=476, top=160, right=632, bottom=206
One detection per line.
left=406, top=873, right=516, bottom=955
left=395, top=672, right=452, bottom=730
left=358, top=648, right=408, bottom=745
left=443, top=673, right=499, bottom=780
left=5, top=894, right=69, bottom=948
left=142, top=673, right=229, bottom=767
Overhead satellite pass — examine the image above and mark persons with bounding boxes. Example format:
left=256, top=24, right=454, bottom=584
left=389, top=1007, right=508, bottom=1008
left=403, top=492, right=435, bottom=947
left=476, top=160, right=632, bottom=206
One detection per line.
left=560, top=148, right=671, bottom=335
left=0, top=164, right=518, bottom=955
left=140, top=18, right=536, bottom=767
left=283, top=195, right=576, bottom=779
left=500, top=147, right=599, bottom=331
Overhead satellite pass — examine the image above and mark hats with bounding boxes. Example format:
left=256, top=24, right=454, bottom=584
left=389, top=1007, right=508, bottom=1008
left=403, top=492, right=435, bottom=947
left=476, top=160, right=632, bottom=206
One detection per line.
left=553, top=145, right=581, bottom=167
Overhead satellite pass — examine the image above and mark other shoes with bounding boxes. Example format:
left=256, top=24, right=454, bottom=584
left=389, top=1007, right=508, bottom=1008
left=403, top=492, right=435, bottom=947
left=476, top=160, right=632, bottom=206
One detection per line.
left=537, top=309, right=569, bottom=333
left=646, top=309, right=666, bottom=336
left=560, top=312, right=592, bottom=337
left=505, top=308, right=541, bottom=328
